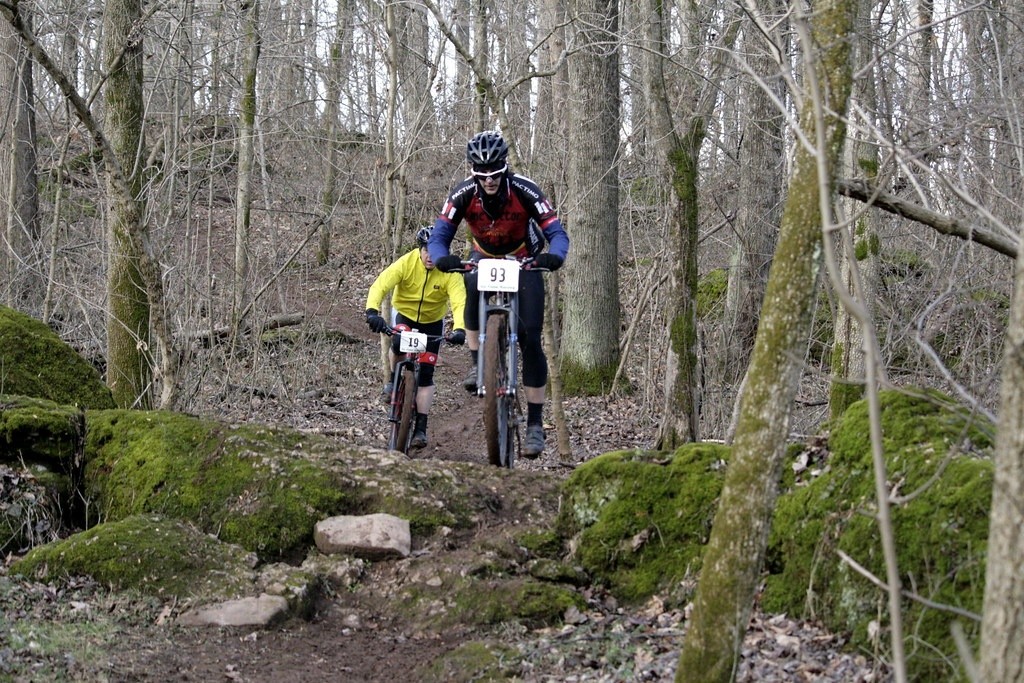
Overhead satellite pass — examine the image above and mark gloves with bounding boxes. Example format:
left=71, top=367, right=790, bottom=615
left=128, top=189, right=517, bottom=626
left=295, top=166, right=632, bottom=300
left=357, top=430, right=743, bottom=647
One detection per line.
left=366, top=308, right=386, bottom=333
left=449, top=329, right=466, bottom=346
left=536, top=254, right=562, bottom=271
left=435, top=255, right=464, bottom=273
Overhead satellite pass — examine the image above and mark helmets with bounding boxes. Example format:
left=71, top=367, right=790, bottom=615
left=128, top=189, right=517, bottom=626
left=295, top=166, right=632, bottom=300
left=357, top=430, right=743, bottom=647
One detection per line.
left=467, top=131, right=509, bottom=164
left=416, top=226, right=435, bottom=244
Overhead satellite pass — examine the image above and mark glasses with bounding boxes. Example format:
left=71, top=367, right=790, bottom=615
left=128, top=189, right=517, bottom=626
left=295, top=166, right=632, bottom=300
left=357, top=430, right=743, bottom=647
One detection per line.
left=470, top=164, right=508, bottom=181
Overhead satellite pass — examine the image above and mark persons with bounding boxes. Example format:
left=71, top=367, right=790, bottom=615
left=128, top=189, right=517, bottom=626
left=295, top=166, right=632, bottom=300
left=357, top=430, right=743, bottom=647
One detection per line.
left=429, top=129, right=570, bottom=459
left=366, top=224, right=469, bottom=451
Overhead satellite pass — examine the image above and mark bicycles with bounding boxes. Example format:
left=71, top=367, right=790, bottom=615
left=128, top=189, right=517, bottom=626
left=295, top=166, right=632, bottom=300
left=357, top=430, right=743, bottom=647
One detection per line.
left=372, top=322, right=457, bottom=452
left=443, top=252, right=551, bottom=470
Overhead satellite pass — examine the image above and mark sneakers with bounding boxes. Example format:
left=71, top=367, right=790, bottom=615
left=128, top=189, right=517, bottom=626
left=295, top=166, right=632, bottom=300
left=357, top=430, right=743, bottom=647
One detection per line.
left=384, top=383, right=393, bottom=395
left=524, top=425, right=546, bottom=454
left=411, top=430, right=427, bottom=446
left=462, top=365, right=478, bottom=391
